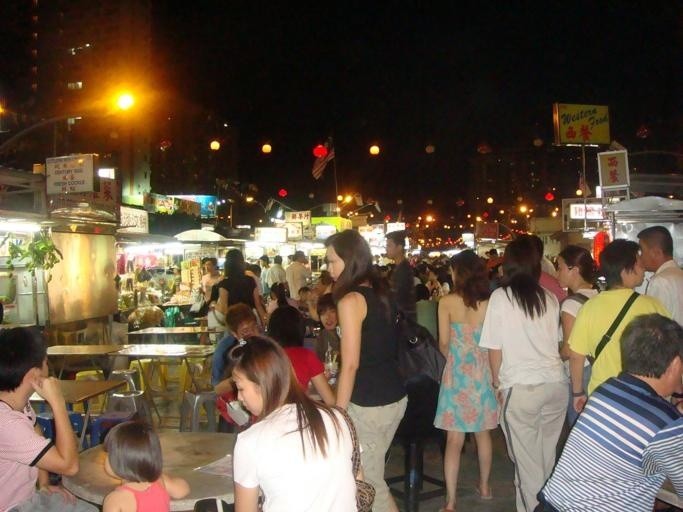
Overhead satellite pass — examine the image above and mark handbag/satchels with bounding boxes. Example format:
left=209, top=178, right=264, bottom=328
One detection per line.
left=566, top=360, right=592, bottom=428
left=395, top=318, right=446, bottom=393
left=189, top=299, right=208, bottom=318
left=355, top=479, right=376, bottom=512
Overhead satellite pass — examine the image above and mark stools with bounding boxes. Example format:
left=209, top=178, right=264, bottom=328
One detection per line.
left=383, top=432, right=447, bottom=512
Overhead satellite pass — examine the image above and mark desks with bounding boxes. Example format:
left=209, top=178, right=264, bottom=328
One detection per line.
left=27, top=327, right=264, bottom=512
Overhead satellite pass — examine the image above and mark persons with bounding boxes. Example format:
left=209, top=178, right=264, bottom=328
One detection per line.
left=0, top=325, right=100, bottom=512
left=101, top=419, right=192, bottom=511
left=172, top=267, right=181, bottom=292
left=194, top=227, right=683, bottom=512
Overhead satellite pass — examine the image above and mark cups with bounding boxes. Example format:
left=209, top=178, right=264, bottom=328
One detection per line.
left=323, top=351, right=340, bottom=372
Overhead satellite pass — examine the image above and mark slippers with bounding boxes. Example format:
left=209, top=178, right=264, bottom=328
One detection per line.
left=438, top=507, right=454, bottom=512
left=474, top=481, right=493, bottom=501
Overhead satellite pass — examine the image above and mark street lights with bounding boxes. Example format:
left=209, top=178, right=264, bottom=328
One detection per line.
left=1, top=86, right=138, bottom=153
